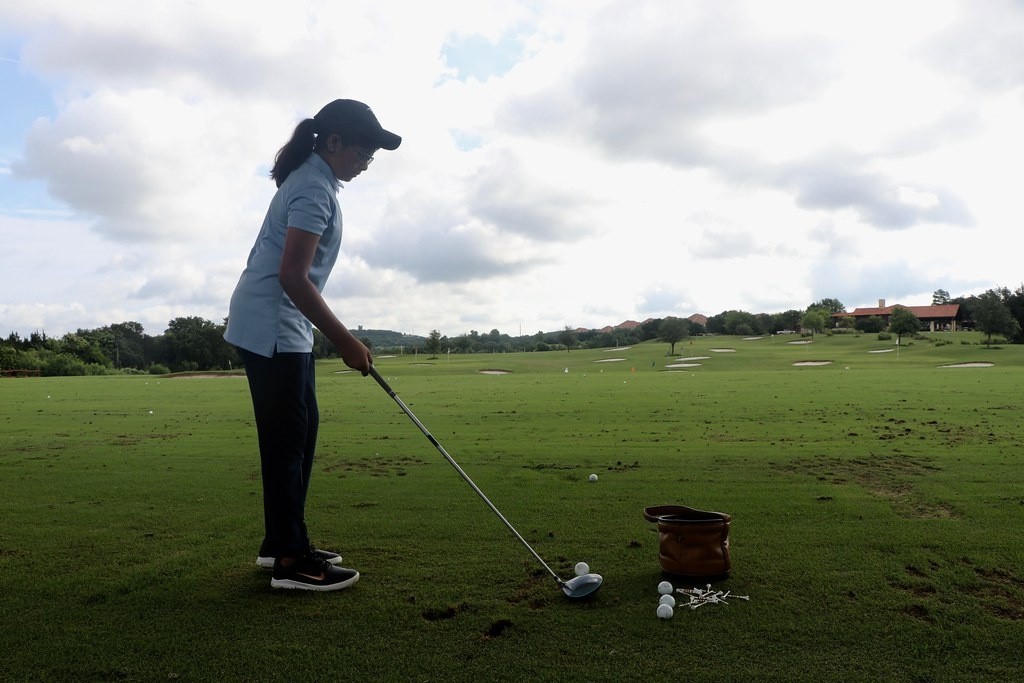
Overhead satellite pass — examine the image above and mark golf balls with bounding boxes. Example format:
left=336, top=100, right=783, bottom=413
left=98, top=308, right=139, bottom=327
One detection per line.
left=658, top=581, right=673, bottom=595
left=590, top=474, right=598, bottom=481
left=574, top=561, right=590, bottom=575
left=657, top=595, right=675, bottom=618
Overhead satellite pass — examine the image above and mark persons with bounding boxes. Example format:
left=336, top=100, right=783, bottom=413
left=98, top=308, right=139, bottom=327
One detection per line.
left=222, top=98, right=402, bottom=590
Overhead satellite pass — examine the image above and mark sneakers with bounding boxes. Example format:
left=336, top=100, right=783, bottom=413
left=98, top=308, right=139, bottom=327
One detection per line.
left=256, top=537, right=342, bottom=568
left=270, top=545, right=359, bottom=591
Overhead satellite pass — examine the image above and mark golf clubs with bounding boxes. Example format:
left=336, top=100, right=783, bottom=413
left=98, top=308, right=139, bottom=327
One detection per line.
left=369, top=363, right=605, bottom=600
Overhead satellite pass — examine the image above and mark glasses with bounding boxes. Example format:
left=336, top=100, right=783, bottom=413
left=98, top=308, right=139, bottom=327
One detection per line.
left=341, top=134, right=374, bottom=166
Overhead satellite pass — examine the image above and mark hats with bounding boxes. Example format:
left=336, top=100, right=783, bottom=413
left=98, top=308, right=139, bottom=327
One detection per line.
left=314, top=99, right=402, bottom=150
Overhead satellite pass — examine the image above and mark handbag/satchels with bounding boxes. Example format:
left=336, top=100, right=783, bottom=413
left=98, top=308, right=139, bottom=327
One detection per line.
left=643, top=504, right=732, bottom=580
